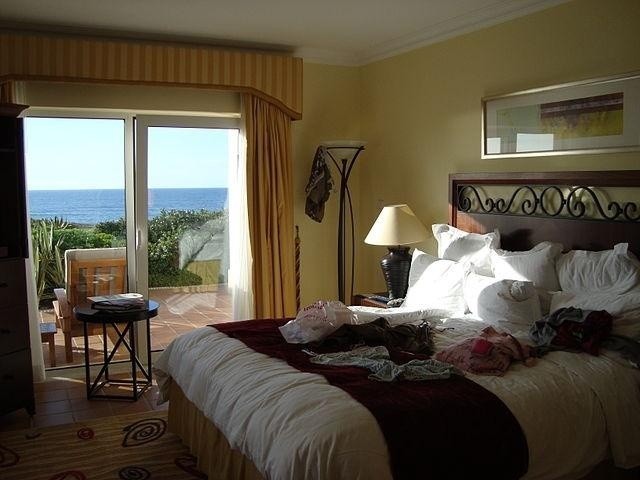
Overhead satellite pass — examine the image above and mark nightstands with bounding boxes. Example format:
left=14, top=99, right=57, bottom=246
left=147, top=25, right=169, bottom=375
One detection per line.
left=361, top=296, right=388, bottom=308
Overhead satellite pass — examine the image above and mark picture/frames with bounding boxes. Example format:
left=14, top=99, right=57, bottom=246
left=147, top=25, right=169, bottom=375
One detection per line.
left=481, top=70, right=640, bottom=159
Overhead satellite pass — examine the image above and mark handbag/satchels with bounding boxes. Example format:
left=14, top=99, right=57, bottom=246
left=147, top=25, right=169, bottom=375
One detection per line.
left=392, top=324, right=431, bottom=355
left=86, top=292, right=145, bottom=313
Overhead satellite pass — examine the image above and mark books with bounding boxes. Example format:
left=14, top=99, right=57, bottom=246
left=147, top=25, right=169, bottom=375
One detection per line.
left=86, top=292, right=146, bottom=313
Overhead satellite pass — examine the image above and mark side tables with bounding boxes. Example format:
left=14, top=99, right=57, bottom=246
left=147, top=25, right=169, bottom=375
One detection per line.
left=41, top=323, right=57, bottom=367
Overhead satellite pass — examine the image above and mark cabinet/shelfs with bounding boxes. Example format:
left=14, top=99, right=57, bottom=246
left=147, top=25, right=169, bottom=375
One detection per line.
left=0, top=258, right=39, bottom=417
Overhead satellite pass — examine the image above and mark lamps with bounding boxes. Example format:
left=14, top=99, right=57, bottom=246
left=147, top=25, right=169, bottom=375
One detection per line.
left=364, top=205, right=432, bottom=302
left=320, top=139, right=366, bottom=306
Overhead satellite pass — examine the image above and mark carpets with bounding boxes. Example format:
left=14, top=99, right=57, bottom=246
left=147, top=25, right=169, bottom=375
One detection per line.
left=0, top=410, right=211, bottom=480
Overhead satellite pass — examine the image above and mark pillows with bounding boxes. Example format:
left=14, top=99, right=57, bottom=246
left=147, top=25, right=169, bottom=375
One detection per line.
left=64, top=247, right=127, bottom=300
left=401, top=224, right=640, bottom=345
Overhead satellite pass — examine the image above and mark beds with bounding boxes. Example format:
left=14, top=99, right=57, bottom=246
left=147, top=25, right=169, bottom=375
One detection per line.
left=152, top=173, right=640, bottom=480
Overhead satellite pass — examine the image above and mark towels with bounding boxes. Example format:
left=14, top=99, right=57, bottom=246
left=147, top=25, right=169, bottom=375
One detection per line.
left=305, top=144, right=334, bottom=222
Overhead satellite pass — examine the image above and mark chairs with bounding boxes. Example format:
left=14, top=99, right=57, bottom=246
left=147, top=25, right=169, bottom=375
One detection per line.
left=52, top=259, right=127, bottom=363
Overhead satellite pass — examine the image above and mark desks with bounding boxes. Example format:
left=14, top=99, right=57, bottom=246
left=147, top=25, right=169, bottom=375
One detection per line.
left=73, top=301, right=159, bottom=402
